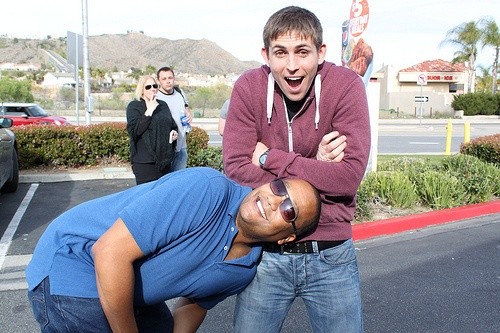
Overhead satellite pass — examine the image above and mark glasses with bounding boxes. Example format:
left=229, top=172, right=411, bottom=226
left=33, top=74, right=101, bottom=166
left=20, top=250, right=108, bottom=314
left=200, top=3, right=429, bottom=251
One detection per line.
left=270, top=178, right=297, bottom=234
left=144, top=84, right=158, bottom=90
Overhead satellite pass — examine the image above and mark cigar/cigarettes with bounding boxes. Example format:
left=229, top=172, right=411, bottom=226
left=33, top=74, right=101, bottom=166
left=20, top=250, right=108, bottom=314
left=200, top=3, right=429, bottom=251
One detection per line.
left=153, top=94, right=157, bottom=96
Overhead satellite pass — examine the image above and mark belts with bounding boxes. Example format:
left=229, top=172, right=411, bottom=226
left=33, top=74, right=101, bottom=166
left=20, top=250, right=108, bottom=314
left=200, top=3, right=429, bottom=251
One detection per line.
left=262, top=241, right=345, bottom=253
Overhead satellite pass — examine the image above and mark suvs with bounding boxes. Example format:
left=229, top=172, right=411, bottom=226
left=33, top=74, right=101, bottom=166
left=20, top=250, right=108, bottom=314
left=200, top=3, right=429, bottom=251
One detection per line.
left=0, top=103, right=69, bottom=127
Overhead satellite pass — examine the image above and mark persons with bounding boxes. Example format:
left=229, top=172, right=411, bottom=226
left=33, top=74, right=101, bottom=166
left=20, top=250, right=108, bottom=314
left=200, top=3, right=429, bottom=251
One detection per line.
left=218, top=99, right=230, bottom=135
left=126, top=75, right=178, bottom=185
left=156, top=67, right=192, bottom=175
left=24, top=166, right=321, bottom=333
left=222, top=5, right=371, bottom=333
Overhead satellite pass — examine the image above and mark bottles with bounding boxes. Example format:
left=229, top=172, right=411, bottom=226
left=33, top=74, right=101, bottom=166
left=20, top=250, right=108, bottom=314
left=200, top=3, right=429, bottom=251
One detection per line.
left=180, top=109, right=192, bottom=134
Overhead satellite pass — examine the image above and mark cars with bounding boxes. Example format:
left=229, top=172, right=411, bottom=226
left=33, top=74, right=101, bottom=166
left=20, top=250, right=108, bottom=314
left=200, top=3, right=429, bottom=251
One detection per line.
left=0, top=118, right=20, bottom=193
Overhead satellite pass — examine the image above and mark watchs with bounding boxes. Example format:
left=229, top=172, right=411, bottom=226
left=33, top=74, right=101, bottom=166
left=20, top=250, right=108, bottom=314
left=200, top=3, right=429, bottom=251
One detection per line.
left=259, top=148, right=271, bottom=165
